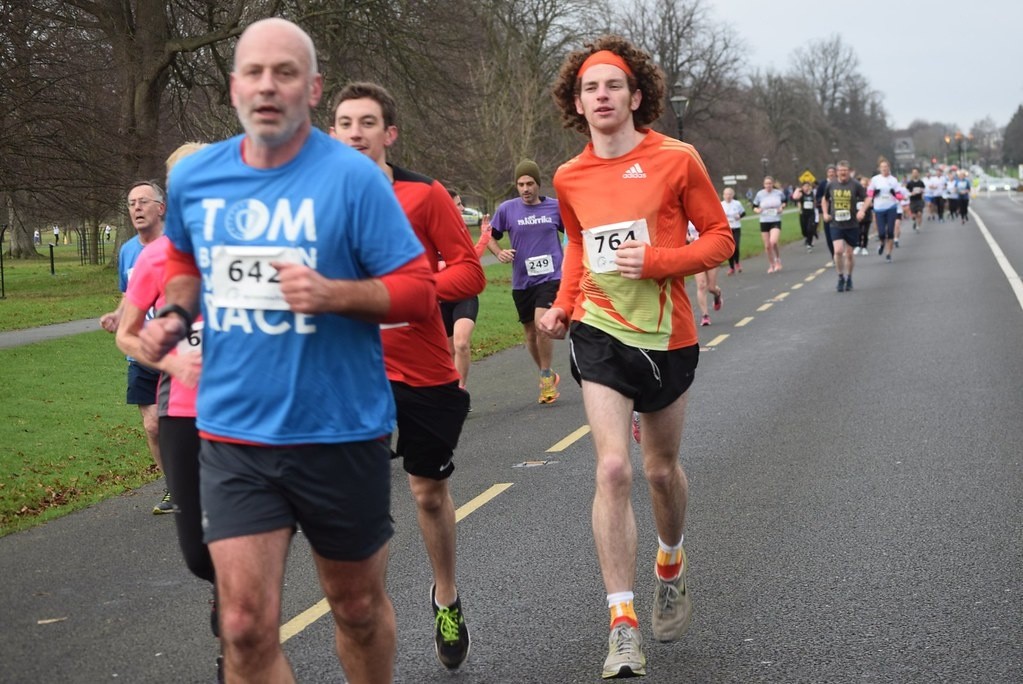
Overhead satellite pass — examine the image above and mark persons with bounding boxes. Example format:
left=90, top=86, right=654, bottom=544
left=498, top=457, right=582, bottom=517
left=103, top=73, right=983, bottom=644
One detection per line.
left=753, top=176, right=786, bottom=274
left=439, top=189, right=491, bottom=412
left=34, top=228, right=40, bottom=245
left=100, top=143, right=222, bottom=684
left=794, top=157, right=970, bottom=262
left=822, top=160, right=871, bottom=292
left=329, top=82, right=487, bottom=674
left=489, top=157, right=564, bottom=403
left=53, top=225, right=59, bottom=246
left=138, top=19, right=434, bottom=684
left=686, top=220, right=722, bottom=326
left=721, top=187, right=745, bottom=275
left=538, top=41, right=733, bottom=679
left=106, top=225, right=111, bottom=241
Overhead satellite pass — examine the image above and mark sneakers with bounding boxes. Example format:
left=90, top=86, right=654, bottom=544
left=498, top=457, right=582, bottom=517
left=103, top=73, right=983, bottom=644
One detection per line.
left=651, top=544, right=691, bottom=643
left=537, top=368, right=561, bottom=405
left=601, top=622, right=647, bottom=679
left=152, top=487, right=175, bottom=514
left=713, top=286, right=723, bottom=311
left=428, top=582, right=471, bottom=676
left=699, top=314, right=712, bottom=326
left=631, top=410, right=642, bottom=444
left=208, top=586, right=221, bottom=640
left=767, top=262, right=783, bottom=274
left=846, top=281, right=852, bottom=291
left=837, top=277, right=845, bottom=292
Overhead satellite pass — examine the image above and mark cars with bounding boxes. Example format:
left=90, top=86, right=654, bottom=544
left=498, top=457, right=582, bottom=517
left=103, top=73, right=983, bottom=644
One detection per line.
left=461, top=208, right=489, bottom=226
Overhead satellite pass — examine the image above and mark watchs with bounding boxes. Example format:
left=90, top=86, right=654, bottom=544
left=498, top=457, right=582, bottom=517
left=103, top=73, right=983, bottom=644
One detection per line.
left=156, top=304, right=191, bottom=333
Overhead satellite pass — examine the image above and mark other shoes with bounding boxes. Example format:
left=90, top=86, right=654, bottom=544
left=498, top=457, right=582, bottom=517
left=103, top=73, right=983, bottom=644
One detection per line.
left=885, top=254, right=891, bottom=263
left=726, top=269, right=735, bottom=275
left=861, top=248, right=869, bottom=256
left=736, top=265, right=742, bottom=273
left=853, top=246, right=861, bottom=255
left=877, top=243, right=884, bottom=254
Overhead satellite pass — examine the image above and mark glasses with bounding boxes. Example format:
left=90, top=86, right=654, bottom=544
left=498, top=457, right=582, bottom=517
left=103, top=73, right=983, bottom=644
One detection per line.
left=127, top=199, right=163, bottom=205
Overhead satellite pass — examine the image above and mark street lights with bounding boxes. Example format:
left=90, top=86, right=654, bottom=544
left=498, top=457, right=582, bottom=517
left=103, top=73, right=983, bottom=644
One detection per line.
left=669, top=82, right=688, bottom=141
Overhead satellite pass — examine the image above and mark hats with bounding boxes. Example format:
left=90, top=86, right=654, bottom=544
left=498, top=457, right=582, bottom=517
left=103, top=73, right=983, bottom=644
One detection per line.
left=824, top=164, right=836, bottom=172
left=763, top=175, right=775, bottom=181
left=511, top=157, right=542, bottom=188
